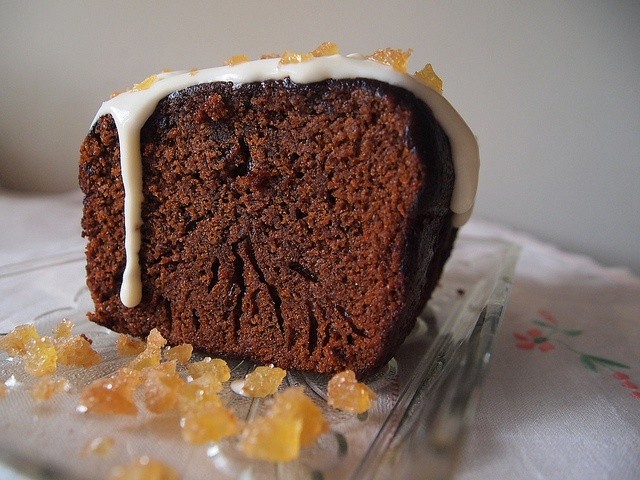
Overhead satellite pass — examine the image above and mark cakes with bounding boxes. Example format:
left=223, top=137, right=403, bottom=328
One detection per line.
left=76, top=53, right=479, bottom=378
